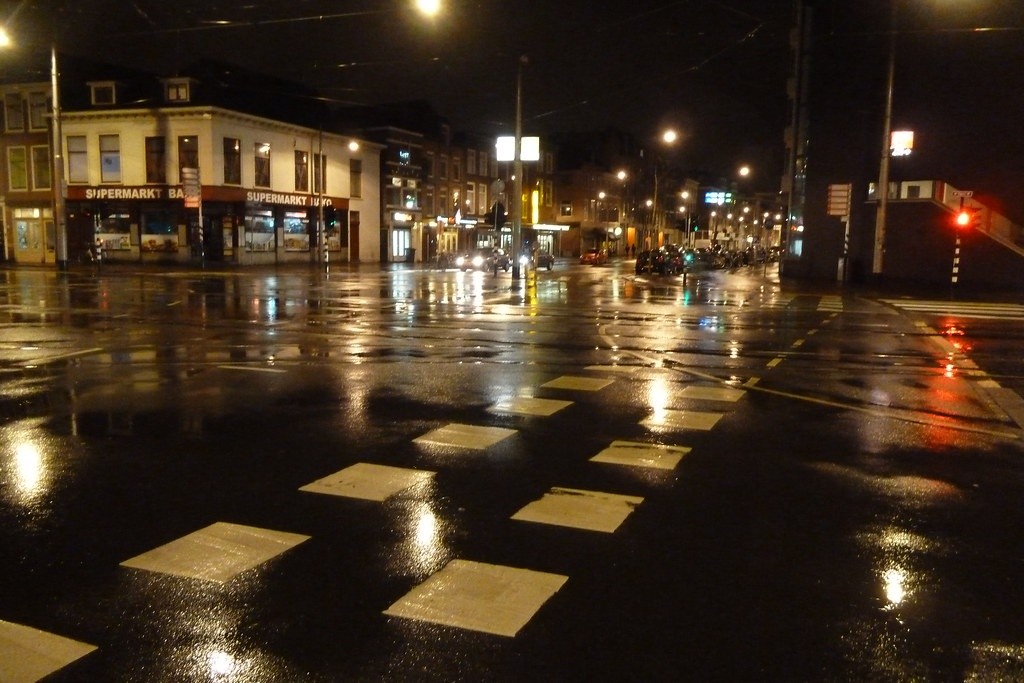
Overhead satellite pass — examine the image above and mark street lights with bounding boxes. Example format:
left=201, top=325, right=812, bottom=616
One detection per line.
left=599, top=192, right=609, bottom=246
left=0, top=27, right=66, bottom=270
left=319, top=125, right=359, bottom=263
left=725, top=206, right=732, bottom=237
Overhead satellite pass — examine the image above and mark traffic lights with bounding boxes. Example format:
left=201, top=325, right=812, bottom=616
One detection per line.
left=497, top=202, right=507, bottom=230
left=325, top=206, right=337, bottom=228
left=956, top=212, right=970, bottom=227
left=966, top=207, right=983, bottom=232
left=484, top=202, right=496, bottom=229
left=690, top=217, right=698, bottom=232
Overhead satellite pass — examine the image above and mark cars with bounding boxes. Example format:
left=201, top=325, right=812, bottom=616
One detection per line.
left=509, top=249, right=555, bottom=271
left=459, top=246, right=509, bottom=272
left=635, top=246, right=780, bottom=276
left=580, top=248, right=608, bottom=264
